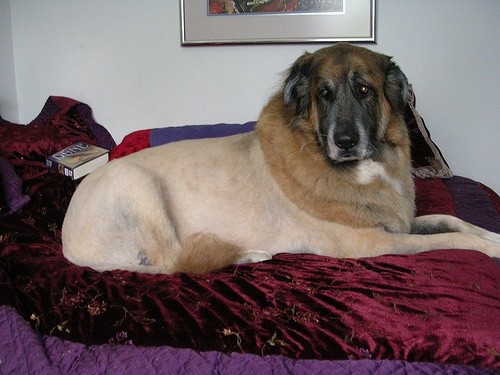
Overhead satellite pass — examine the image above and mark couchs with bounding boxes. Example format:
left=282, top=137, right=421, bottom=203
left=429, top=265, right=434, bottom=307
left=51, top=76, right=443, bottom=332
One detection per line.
left=0, top=93, right=499, bottom=374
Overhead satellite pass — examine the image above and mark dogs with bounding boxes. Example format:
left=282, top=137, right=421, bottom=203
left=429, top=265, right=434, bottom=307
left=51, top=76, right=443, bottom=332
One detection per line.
left=60, top=43, right=500, bottom=276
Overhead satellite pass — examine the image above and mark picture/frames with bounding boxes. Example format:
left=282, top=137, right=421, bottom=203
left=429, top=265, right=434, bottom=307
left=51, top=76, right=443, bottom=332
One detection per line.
left=179, top=0, right=378, bottom=46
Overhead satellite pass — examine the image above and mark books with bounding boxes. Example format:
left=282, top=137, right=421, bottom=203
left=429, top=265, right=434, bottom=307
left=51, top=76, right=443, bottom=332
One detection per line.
left=45, top=140, right=109, bottom=182
